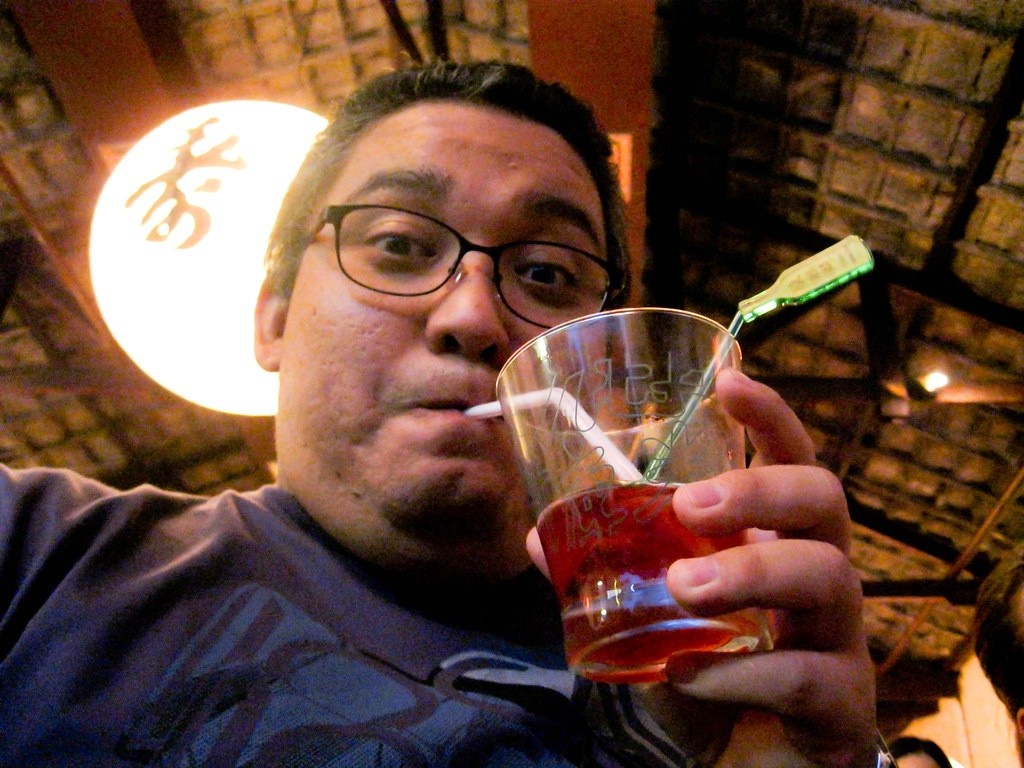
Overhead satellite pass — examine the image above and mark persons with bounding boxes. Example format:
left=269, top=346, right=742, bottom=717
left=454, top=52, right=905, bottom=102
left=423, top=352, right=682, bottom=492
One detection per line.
left=968, top=541, right=1024, bottom=768
left=887, top=735, right=953, bottom=768
left=0, top=61, right=880, bottom=768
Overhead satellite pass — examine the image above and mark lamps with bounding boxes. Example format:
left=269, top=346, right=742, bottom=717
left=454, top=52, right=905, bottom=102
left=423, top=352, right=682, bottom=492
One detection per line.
left=82, top=99, right=332, bottom=419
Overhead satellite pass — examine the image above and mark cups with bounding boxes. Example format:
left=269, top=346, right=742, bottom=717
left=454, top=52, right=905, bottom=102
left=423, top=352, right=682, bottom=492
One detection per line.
left=494, top=304, right=776, bottom=688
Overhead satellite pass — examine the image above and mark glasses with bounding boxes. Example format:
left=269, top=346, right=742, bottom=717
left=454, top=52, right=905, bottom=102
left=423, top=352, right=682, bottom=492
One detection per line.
left=302, top=202, right=626, bottom=331
